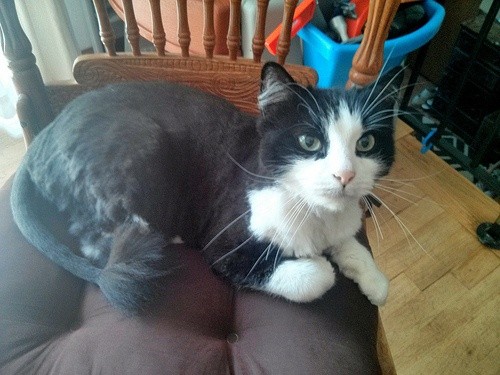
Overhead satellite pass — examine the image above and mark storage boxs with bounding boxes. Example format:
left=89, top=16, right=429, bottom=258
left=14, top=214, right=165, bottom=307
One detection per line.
left=296, top=0, right=446, bottom=92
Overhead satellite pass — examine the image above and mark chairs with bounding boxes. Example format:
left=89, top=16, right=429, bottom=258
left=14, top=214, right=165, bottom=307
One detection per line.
left=0, top=0, right=398, bottom=375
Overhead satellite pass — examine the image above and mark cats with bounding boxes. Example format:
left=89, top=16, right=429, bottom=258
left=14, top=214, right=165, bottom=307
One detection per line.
left=10, top=61, right=405, bottom=317
